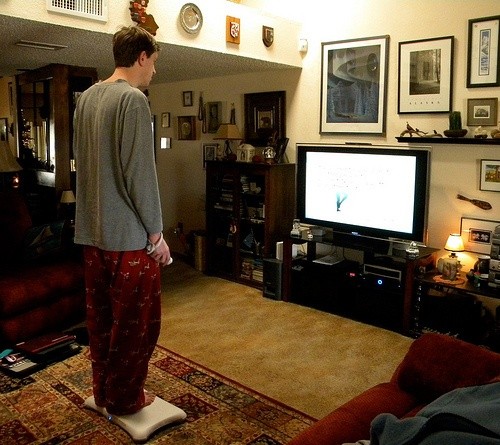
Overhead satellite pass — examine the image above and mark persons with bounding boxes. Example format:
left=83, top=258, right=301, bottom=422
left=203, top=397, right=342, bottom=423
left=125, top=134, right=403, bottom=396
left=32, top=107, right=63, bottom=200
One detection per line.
left=72, top=26, right=171, bottom=416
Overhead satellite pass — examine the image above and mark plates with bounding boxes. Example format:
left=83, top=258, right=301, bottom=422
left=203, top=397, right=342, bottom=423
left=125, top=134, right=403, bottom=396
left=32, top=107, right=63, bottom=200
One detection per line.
left=178, top=3, right=204, bottom=35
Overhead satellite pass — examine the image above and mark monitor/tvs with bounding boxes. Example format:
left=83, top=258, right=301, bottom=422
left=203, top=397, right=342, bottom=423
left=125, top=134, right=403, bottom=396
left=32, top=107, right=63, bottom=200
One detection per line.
left=296, top=143, right=431, bottom=249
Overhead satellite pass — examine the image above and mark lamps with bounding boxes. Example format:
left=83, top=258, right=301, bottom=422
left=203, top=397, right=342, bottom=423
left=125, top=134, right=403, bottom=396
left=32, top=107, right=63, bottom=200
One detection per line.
left=0, top=141, right=23, bottom=192
left=444, top=233, right=465, bottom=259
left=212, top=123, right=242, bottom=159
left=59, top=190, right=76, bottom=205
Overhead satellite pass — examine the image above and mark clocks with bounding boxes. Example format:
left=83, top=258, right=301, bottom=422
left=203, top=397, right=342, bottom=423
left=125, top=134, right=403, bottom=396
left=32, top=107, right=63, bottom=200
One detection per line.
left=179, top=3, right=204, bottom=33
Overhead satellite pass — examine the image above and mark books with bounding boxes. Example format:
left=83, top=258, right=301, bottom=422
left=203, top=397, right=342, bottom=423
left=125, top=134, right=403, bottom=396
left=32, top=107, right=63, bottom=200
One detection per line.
left=241, top=258, right=263, bottom=282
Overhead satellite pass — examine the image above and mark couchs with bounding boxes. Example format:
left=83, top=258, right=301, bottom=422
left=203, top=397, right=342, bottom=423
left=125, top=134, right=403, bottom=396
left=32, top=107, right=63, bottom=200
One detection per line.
left=281, top=333, right=500, bottom=445
left=0, top=192, right=87, bottom=342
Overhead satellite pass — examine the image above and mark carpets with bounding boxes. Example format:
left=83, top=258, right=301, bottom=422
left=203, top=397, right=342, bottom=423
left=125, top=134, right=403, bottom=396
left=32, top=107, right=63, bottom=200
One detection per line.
left=0, top=328, right=321, bottom=444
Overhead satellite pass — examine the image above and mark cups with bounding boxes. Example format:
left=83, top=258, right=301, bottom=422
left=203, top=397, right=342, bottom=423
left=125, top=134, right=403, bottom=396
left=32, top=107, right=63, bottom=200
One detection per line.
left=477, top=254, right=490, bottom=278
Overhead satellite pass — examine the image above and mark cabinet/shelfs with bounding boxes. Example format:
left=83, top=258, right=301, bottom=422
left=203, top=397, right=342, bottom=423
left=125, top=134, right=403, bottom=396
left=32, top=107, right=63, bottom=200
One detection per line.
left=289, top=229, right=439, bottom=331
left=205, top=160, right=296, bottom=291
left=411, top=266, right=500, bottom=355
left=396, top=136, right=500, bottom=145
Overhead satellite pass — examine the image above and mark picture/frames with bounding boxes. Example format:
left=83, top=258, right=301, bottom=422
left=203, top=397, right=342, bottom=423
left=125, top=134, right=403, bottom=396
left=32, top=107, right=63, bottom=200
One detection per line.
left=205, top=102, right=222, bottom=133
left=203, top=145, right=217, bottom=169
left=467, top=98, right=498, bottom=125
left=183, top=91, right=192, bottom=106
left=398, top=37, right=454, bottom=114
left=480, top=159, right=500, bottom=192
left=161, top=112, right=170, bottom=128
left=460, top=217, right=500, bottom=257
left=243, top=91, right=286, bottom=146
left=467, top=16, right=500, bottom=88
left=320, top=33, right=391, bottom=137
left=177, top=116, right=196, bottom=140
